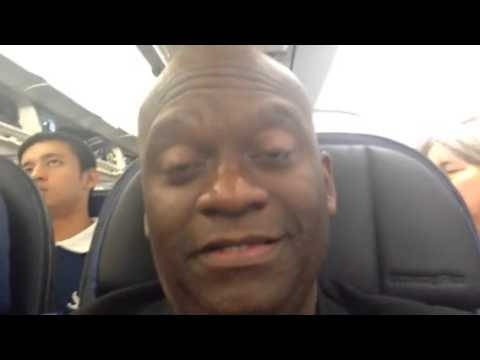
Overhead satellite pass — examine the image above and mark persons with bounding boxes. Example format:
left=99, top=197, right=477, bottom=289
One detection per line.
left=18, top=132, right=100, bottom=314
left=66, top=45, right=472, bottom=315
left=417, top=116, right=480, bottom=243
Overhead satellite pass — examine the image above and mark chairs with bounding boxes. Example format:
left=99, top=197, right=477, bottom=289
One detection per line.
left=75, top=131, right=480, bottom=315
left=0, top=154, right=53, bottom=315
